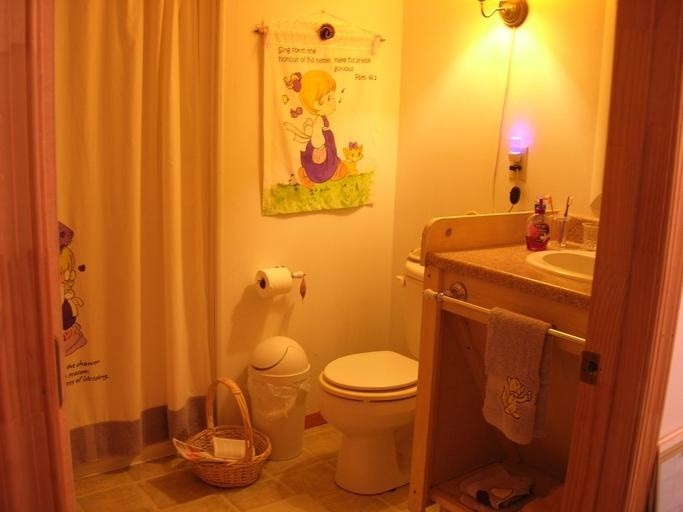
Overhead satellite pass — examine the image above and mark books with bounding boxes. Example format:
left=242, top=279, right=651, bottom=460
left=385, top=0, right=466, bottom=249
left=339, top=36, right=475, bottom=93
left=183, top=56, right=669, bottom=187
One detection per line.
left=172, top=436, right=238, bottom=464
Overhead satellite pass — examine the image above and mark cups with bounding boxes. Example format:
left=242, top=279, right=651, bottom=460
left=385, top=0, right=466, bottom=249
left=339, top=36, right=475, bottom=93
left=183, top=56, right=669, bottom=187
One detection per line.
left=548, top=214, right=569, bottom=249
left=581, top=222, right=598, bottom=252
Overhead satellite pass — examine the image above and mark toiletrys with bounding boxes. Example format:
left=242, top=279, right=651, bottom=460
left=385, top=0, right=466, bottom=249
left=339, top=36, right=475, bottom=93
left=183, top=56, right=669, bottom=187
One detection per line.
left=524, top=196, right=552, bottom=252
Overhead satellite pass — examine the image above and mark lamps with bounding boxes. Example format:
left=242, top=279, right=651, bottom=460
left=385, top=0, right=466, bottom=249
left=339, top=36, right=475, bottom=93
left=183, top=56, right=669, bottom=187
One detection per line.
left=506, top=134, right=523, bottom=168
left=478, top=0, right=528, bottom=28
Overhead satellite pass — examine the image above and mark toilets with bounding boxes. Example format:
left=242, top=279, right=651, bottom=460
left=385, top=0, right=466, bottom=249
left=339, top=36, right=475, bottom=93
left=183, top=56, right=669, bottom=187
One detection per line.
left=320, top=246, right=425, bottom=495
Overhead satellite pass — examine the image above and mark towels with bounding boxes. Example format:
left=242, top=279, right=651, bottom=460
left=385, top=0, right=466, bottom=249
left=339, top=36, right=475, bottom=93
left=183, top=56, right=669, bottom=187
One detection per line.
left=479, top=304, right=555, bottom=448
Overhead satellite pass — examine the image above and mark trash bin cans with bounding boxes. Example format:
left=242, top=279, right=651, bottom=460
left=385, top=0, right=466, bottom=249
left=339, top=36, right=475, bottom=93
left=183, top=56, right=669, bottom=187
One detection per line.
left=247, top=336, right=310, bottom=461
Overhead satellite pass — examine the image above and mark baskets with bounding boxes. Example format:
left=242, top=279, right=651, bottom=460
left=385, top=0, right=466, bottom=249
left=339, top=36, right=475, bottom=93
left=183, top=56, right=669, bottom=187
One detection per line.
left=182, top=378, right=273, bottom=488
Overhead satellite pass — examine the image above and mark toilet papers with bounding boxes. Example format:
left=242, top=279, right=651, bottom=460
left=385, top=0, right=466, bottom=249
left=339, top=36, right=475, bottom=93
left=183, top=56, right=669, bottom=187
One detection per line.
left=256, top=267, right=294, bottom=298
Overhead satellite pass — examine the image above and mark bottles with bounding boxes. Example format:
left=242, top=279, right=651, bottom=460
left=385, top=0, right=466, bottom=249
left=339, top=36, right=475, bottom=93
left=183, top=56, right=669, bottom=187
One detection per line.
left=526, top=197, right=550, bottom=251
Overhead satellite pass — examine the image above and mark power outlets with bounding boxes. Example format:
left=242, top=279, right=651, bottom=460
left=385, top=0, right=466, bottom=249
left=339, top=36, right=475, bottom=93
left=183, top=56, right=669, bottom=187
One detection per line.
left=507, top=146, right=529, bottom=184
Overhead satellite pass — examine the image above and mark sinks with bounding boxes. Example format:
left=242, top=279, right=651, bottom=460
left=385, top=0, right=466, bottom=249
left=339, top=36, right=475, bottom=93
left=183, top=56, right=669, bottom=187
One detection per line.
left=527, top=248, right=596, bottom=282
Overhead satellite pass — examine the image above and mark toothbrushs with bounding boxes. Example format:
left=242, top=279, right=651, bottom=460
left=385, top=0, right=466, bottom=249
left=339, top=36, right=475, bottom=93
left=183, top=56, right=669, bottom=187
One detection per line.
left=546, top=194, right=560, bottom=243
left=559, top=194, right=575, bottom=244
